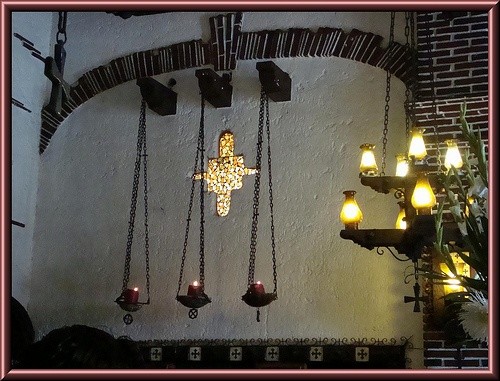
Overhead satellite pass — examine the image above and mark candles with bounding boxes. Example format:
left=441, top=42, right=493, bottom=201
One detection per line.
left=255, top=281, right=265, bottom=295
left=187, top=281, right=201, bottom=297
left=124, top=288, right=139, bottom=303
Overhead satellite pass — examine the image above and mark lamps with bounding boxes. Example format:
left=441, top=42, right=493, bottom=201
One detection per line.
left=339, top=12, right=464, bottom=313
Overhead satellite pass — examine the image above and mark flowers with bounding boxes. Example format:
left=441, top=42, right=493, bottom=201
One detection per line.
left=433, top=99, right=489, bottom=346
